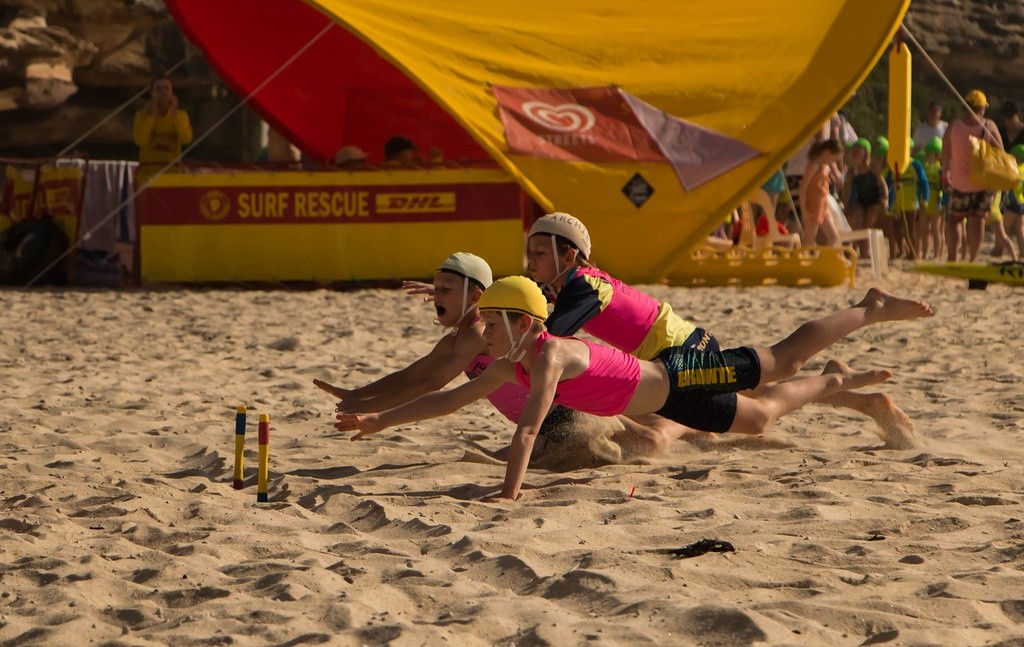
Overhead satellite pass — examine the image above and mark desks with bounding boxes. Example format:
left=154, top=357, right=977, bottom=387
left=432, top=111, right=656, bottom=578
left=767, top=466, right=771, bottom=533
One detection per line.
left=133, top=161, right=528, bottom=283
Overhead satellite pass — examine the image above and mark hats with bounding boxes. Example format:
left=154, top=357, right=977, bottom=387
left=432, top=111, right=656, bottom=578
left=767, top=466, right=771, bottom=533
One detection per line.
left=436, top=251, right=494, bottom=289
left=844, top=135, right=942, bottom=158
left=335, top=148, right=368, bottom=165
left=384, top=136, right=417, bottom=162
left=479, top=276, right=549, bottom=324
left=529, top=212, right=591, bottom=260
left=966, top=90, right=989, bottom=107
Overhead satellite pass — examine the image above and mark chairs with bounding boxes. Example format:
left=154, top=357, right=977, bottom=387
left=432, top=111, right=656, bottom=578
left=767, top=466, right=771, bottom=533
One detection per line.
left=825, top=194, right=889, bottom=281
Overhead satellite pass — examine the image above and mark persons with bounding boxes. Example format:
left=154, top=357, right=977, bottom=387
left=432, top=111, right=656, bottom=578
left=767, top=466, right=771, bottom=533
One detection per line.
left=334, top=137, right=417, bottom=169
left=132, top=80, right=192, bottom=164
left=313, top=211, right=933, bottom=505
left=723, top=87, right=1024, bottom=263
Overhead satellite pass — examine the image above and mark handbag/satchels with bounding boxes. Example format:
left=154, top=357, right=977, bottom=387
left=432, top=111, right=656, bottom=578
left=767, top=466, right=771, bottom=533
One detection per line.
left=967, top=118, right=1019, bottom=191
